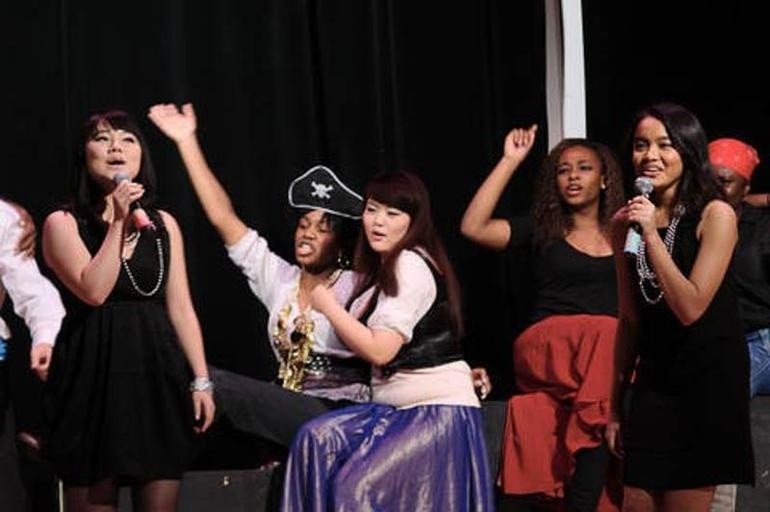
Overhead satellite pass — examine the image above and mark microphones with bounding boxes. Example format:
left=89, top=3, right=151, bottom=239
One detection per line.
left=114, top=172, right=154, bottom=241
left=622, top=175, right=654, bottom=260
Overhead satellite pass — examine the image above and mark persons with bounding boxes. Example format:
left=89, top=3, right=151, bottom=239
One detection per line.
left=459, top=122, right=626, bottom=510
left=40, top=110, right=216, bottom=511
left=704, top=137, right=770, bottom=401
left=0, top=199, right=68, bottom=510
left=7, top=202, right=39, bottom=265
left=146, top=103, right=496, bottom=463
left=618, top=101, right=759, bottom=512
left=277, top=167, right=495, bottom=512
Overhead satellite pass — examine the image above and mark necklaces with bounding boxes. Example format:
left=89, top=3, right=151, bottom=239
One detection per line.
left=634, top=209, right=682, bottom=308
left=123, top=231, right=165, bottom=298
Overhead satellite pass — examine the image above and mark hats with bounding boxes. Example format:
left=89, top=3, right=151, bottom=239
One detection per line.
left=288, top=165, right=364, bottom=220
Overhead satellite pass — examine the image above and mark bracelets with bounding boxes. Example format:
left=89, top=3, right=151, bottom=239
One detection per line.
left=189, top=377, right=216, bottom=392
